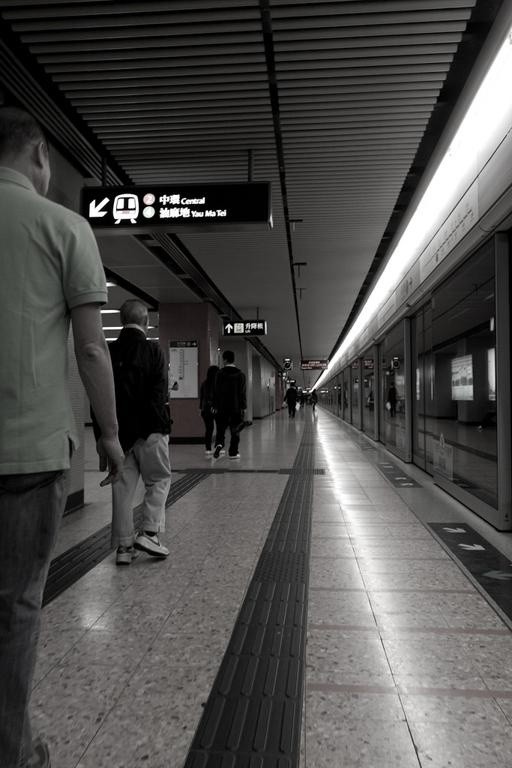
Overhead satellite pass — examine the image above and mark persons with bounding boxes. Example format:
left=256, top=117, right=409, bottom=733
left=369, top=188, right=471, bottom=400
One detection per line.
left=0, top=110, right=127, bottom=768
left=283, top=383, right=318, bottom=418
left=385, top=382, right=396, bottom=417
left=211, top=350, right=246, bottom=459
left=108, top=300, right=174, bottom=565
left=89, top=405, right=111, bottom=472
left=198, top=366, right=225, bottom=455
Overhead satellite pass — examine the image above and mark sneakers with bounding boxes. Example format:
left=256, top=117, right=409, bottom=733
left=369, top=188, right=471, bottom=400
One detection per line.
left=27, top=737, right=51, bottom=767
left=206, top=445, right=240, bottom=459
left=116, top=546, right=139, bottom=563
left=133, top=532, right=170, bottom=556
